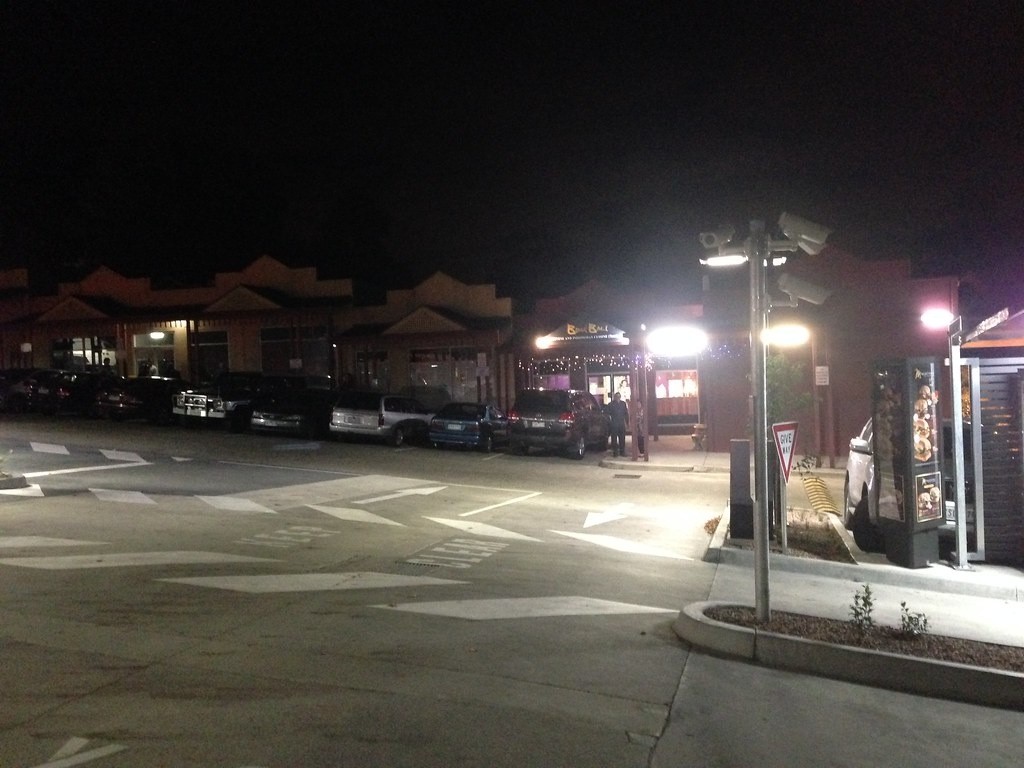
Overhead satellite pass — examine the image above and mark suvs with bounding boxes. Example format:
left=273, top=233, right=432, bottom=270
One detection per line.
left=507, top=389, right=612, bottom=460
left=843, top=416, right=983, bottom=563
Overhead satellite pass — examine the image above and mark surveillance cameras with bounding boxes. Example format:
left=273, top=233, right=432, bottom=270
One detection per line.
left=778, top=212, right=834, bottom=256
left=698, top=223, right=736, bottom=247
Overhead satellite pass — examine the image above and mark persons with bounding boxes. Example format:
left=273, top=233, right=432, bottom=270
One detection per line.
left=214, top=363, right=227, bottom=374
left=140, top=359, right=180, bottom=379
left=103, top=359, right=112, bottom=371
left=620, top=381, right=630, bottom=406
left=606, top=392, right=629, bottom=457
left=637, top=399, right=645, bottom=458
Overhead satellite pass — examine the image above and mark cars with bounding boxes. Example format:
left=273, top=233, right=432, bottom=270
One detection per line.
left=328, top=393, right=437, bottom=447
left=429, top=402, right=512, bottom=453
left=0, top=370, right=328, bottom=441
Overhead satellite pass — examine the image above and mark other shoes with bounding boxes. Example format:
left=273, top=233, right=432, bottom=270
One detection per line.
left=638, top=452, right=645, bottom=458
left=613, top=452, right=619, bottom=457
left=620, top=452, right=628, bottom=457
